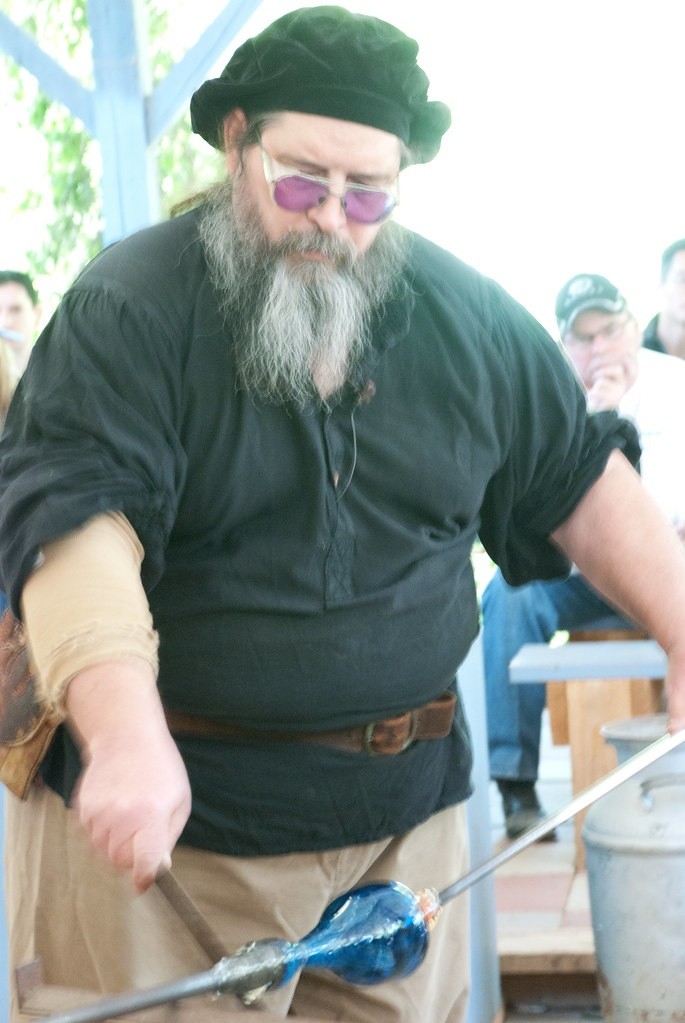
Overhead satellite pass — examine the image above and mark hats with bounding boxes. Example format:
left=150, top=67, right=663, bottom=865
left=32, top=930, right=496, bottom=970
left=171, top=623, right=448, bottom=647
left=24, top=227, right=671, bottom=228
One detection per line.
left=555, top=274, right=624, bottom=337
left=190, top=6, right=452, bottom=164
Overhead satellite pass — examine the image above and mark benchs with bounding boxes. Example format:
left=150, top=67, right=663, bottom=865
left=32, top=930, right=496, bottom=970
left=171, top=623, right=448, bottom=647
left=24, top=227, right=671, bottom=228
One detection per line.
left=509, top=631, right=668, bottom=871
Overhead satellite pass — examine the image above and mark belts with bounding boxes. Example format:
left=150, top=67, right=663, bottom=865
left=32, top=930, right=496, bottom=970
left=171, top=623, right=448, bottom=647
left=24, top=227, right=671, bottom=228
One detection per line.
left=162, top=689, right=456, bottom=760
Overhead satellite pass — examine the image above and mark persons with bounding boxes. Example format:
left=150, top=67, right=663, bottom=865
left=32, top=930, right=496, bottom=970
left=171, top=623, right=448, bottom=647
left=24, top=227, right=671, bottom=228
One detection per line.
left=641, top=239, right=684, bottom=360
left=481, top=273, right=685, bottom=840
left=0, top=270, right=40, bottom=363
left=0, top=5, right=685, bottom=1023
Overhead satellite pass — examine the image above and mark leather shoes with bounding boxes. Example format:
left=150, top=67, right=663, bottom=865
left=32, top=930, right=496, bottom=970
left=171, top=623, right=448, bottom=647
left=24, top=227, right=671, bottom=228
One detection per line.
left=498, top=782, right=555, bottom=840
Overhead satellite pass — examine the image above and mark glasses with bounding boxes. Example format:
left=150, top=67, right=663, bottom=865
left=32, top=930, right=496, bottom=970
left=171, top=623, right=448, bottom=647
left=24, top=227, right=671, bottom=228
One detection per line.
left=248, top=119, right=399, bottom=223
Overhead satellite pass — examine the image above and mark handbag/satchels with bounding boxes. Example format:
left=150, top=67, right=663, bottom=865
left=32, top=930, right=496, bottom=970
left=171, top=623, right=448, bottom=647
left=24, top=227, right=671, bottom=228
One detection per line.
left=0, top=602, right=68, bottom=802
